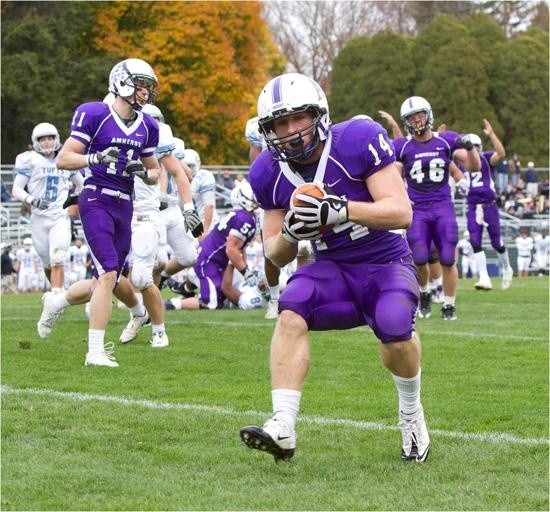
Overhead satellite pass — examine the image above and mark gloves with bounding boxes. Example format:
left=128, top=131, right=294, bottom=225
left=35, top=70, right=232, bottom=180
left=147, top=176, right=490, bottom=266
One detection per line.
left=31, top=198, right=50, bottom=211
left=454, top=178, right=470, bottom=195
left=87, top=147, right=121, bottom=167
left=126, top=160, right=148, bottom=179
left=182, top=210, right=204, bottom=238
left=279, top=211, right=322, bottom=244
left=241, top=267, right=257, bottom=287
left=456, top=133, right=472, bottom=151
left=293, top=193, right=348, bottom=228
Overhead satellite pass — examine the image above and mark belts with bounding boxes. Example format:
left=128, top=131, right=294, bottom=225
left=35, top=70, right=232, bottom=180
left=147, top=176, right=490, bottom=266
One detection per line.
left=85, top=184, right=130, bottom=201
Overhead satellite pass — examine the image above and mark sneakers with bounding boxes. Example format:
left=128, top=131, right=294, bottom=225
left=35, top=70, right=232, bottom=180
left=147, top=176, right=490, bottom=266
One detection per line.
left=37, top=287, right=64, bottom=340
left=397, top=404, right=431, bottom=463
left=263, top=299, right=278, bottom=319
left=441, top=304, right=457, bottom=320
left=239, top=418, right=296, bottom=463
left=158, top=274, right=167, bottom=291
left=84, top=350, right=118, bottom=367
left=119, top=309, right=151, bottom=343
left=167, top=279, right=195, bottom=297
left=151, top=327, right=169, bottom=348
left=474, top=279, right=492, bottom=290
left=418, top=290, right=432, bottom=318
left=430, top=284, right=444, bottom=304
left=501, top=268, right=513, bottom=290
left=164, top=297, right=182, bottom=310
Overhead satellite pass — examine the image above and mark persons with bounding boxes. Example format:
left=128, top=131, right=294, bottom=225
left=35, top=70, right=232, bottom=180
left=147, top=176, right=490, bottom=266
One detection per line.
left=234, top=173, right=254, bottom=201
left=183, top=149, right=219, bottom=241
left=1, top=242, right=17, bottom=296
left=10, top=121, right=85, bottom=296
left=69, top=233, right=92, bottom=285
left=219, top=169, right=236, bottom=192
left=389, top=95, right=483, bottom=322
left=243, top=117, right=313, bottom=322
left=222, top=246, right=269, bottom=313
left=496, top=153, right=550, bottom=219
left=533, top=228, right=549, bottom=278
left=35, top=57, right=163, bottom=369
left=456, top=230, right=478, bottom=278
left=14, top=237, right=41, bottom=294
left=163, top=177, right=255, bottom=312
left=112, top=175, right=170, bottom=350
left=437, top=117, right=514, bottom=292
left=142, top=102, right=199, bottom=291
left=234, top=72, right=431, bottom=466
left=514, top=227, right=534, bottom=278
left=448, top=161, right=470, bottom=199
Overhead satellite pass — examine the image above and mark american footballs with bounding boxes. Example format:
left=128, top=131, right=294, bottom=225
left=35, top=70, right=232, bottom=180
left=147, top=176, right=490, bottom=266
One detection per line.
left=289, top=182, right=334, bottom=239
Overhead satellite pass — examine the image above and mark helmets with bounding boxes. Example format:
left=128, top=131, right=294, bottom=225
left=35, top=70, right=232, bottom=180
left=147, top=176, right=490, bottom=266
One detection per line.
left=108, top=59, right=158, bottom=112
left=184, top=148, right=201, bottom=175
left=141, top=104, right=164, bottom=123
left=31, top=122, right=61, bottom=153
left=255, top=73, right=329, bottom=162
left=229, top=184, right=257, bottom=213
left=461, top=133, right=482, bottom=151
left=237, top=290, right=263, bottom=309
left=399, top=96, right=434, bottom=135
